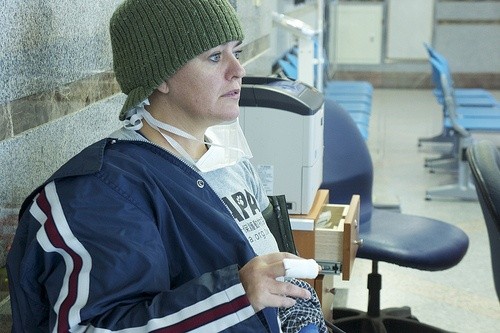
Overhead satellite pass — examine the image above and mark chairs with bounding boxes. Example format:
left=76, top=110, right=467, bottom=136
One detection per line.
left=274, top=35, right=373, bottom=140
left=319, top=98, right=469, bottom=333
left=466, top=138, right=500, bottom=225
left=418, top=41, right=500, bottom=202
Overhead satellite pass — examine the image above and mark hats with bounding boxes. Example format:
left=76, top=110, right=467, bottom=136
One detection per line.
left=109, top=0, right=245, bottom=121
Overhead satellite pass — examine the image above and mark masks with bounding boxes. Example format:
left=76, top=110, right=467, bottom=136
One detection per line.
left=166, top=117, right=254, bottom=173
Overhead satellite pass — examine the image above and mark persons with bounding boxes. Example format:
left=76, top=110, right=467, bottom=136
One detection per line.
left=7, top=1, right=328, bottom=333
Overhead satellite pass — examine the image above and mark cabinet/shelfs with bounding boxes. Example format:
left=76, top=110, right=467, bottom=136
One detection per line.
left=289, top=188, right=363, bottom=315
